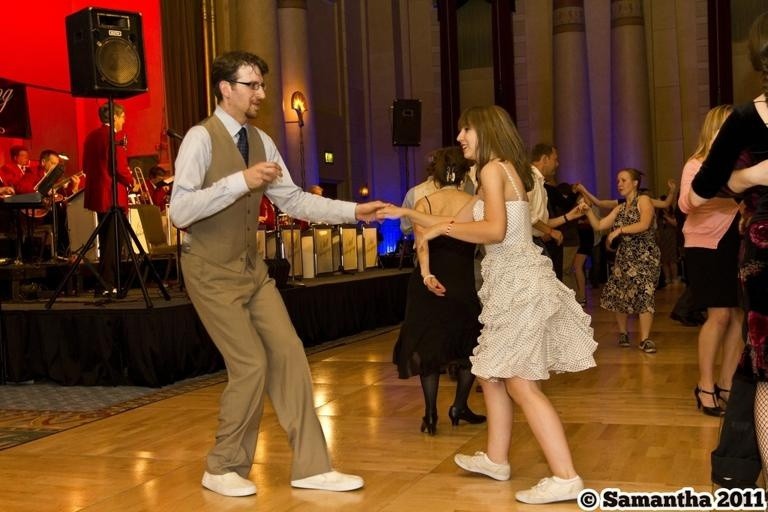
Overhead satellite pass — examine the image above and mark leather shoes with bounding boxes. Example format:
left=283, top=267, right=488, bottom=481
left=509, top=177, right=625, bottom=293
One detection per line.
left=94, top=286, right=131, bottom=299
left=710, top=469, right=768, bottom=502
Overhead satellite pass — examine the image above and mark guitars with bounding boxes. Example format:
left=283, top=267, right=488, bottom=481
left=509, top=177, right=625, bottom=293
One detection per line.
left=21, top=170, right=86, bottom=218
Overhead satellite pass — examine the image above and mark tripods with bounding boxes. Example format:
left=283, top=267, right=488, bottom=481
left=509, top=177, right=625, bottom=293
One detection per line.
left=44, top=99, right=170, bottom=309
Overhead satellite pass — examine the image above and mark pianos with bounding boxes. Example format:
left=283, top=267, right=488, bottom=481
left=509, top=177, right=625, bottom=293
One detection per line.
left=4, top=191, right=41, bottom=208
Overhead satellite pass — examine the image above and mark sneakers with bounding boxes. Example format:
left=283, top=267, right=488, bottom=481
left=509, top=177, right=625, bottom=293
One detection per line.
left=290, top=469, right=365, bottom=492
left=201, top=469, right=257, bottom=498
left=514, top=473, right=586, bottom=504
left=453, top=451, right=512, bottom=482
left=618, top=331, right=632, bottom=347
left=576, top=296, right=587, bottom=307
left=637, top=336, right=657, bottom=353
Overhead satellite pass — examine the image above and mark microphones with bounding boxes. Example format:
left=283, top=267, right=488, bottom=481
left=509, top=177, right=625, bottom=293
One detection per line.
left=167, top=129, right=182, bottom=141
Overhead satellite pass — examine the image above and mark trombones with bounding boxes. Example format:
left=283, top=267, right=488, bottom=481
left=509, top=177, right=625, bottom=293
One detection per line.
left=134, top=166, right=153, bottom=205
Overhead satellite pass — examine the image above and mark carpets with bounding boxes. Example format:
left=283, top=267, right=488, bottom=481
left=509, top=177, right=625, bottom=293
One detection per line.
left=0, top=323, right=402, bottom=450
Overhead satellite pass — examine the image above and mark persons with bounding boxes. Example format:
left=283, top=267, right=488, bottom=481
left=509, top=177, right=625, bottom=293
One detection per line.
left=168, top=50, right=391, bottom=497
left=710, top=159, right=768, bottom=504
left=392, top=142, right=707, bottom=436
left=374, top=104, right=598, bottom=506
left=688, top=11, right=768, bottom=503
left=676, top=104, right=747, bottom=419
left=1, top=102, right=323, bottom=299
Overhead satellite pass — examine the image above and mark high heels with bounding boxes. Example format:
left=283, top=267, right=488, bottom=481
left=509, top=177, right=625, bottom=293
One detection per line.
left=713, top=383, right=730, bottom=406
left=448, top=404, right=487, bottom=427
left=421, top=413, right=439, bottom=436
left=694, top=383, right=728, bottom=417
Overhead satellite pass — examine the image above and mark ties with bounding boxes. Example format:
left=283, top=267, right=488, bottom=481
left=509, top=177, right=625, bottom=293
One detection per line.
left=236, top=126, right=249, bottom=168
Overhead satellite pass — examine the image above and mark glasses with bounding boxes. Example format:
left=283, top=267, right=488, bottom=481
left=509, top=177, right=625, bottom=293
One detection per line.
left=228, top=79, right=267, bottom=91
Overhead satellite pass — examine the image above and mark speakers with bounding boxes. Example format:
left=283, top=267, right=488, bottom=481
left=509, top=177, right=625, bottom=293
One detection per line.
left=392, top=98, right=423, bottom=148
left=65, top=7, right=149, bottom=98
